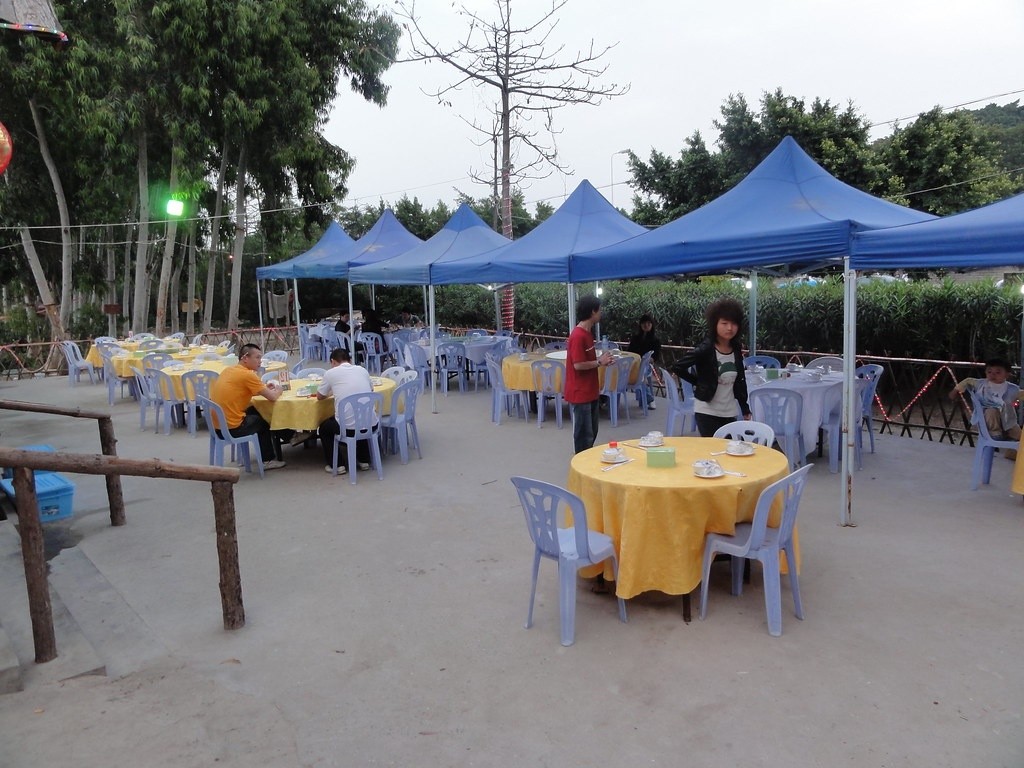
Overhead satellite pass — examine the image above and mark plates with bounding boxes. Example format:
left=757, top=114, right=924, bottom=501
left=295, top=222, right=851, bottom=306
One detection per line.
left=639, top=443, right=662, bottom=447
left=694, top=472, right=724, bottom=478
left=602, top=458, right=627, bottom=464
left=726, top=451, right=754, bottom=455
left=788, top=369, right=800, bottom=372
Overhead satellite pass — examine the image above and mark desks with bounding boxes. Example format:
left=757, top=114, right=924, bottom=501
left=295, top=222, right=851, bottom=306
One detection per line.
left=250, top=377, right=406, bottom=462
left=564, top=437, right=800, bottom=623
left=743, top=368, right=863, bottom=465
left=86, top=339, right=179, bottom=369
left=502, top=350, right=642, bottom=414
left=404, top=335, right=513, bottom=390
left=112, top=346, right=229, bottom=394
left=160, top=360, right=287, bottom=426
left=308, top=326, right=362, bottom=360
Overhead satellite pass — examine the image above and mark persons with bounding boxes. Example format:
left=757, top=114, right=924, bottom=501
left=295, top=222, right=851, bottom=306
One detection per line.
left=622, top=313, right=661, bottom=409
left=670, top=300, right=753, bottom=441
left=392, top=306, right=425, bottom=327
left=563, top=294, right=616, bottom=454
left=316, top=348, right=379, bottom=475
left=358, top=306, right=397, bottom=371
left=210, top=343, right=288, bottom=470
left=334, top=310, right=364, bottom=363
left=948, top=355, right=1024, bottom=442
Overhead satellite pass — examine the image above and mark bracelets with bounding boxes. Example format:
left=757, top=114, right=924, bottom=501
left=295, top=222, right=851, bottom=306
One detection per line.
left=596, top=359, right=601, bottom=367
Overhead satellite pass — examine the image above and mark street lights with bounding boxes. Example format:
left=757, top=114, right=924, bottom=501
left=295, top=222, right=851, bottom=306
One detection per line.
left=611, top=149, right=632, bottom=205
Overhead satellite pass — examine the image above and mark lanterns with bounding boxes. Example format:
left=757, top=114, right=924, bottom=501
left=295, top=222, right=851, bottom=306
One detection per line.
left=0, top=121, right=13, bottom=175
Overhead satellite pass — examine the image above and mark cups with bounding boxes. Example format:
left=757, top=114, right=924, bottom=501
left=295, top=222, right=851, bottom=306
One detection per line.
left=640, top=430, right=663, bottom=444
left=308, top=373, right=317, bottom=380
left=787, top=363, right=796, bottom=370
left=602, top=448, right=625, bottom=460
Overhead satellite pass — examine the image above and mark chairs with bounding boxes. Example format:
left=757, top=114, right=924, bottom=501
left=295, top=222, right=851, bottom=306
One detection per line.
left=298, top=319, right=1024, bottom=490
left=57, top=333, right=814, bottom=647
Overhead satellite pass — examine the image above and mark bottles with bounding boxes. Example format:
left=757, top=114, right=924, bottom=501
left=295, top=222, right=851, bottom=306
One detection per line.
left=602, top=335, right=609, bottom=354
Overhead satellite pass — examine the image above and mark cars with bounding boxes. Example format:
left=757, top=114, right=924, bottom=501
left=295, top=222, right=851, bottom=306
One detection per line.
left=995, top=279, right=1004, bottom=290
left=778, top=277, right=826, bottom=288
left=729, top=278, right=752, bottom=291
left=857, top=275, right=902, bottom=287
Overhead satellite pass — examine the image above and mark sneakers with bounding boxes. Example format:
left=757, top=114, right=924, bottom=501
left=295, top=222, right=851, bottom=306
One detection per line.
left=263, top=460, right=286, bottom=470
left=356, top=461, right=369, bottom=470
left=325, top=464, right=346, bottom=475
left=290, top=432, right=312, bottom=446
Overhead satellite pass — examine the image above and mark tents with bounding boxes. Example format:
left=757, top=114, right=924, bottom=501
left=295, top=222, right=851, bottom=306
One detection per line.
left=255, top=135, right=1024, bottom=528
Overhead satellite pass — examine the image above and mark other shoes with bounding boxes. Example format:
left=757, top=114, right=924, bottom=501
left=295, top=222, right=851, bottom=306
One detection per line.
left=648, top=400, right=656, bottom=409
left=992, top=435, right=1003, bottom=441
left=393, top=359, right=397, bottom=364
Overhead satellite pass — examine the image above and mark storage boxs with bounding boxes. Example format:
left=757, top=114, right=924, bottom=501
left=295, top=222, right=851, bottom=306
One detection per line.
left=0, top=444, right=74, bottom=523
